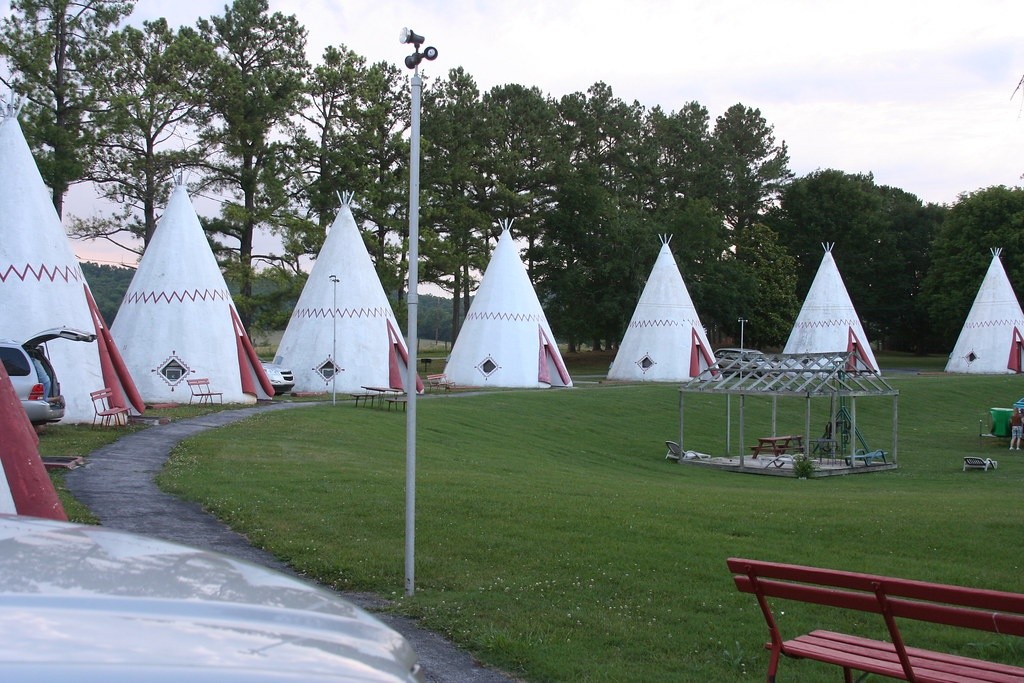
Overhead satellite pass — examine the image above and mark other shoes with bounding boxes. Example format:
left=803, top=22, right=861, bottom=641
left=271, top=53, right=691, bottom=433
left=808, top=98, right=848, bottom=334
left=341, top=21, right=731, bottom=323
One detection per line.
left=1016, top=448, right=1021, bottom=451
left=1009, top=448, right=1015, bottom=450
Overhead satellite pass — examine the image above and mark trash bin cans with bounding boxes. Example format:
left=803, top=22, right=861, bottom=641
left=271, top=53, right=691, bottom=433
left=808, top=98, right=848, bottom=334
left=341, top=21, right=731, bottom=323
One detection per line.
left=989, top=408, right=1013, bottom=436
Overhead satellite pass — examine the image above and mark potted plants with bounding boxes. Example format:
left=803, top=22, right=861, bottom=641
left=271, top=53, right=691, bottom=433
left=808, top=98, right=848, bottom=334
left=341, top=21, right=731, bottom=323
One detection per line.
left=792, top=455, right=816, bottom=479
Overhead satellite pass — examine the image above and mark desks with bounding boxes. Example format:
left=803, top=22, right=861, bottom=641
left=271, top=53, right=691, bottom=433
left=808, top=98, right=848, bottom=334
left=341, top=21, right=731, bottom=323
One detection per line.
left=361, top=386, right=404, bottom=411
left=809, top=438, right=838, bottom=465
left=751, top=435, right=804, bottom=459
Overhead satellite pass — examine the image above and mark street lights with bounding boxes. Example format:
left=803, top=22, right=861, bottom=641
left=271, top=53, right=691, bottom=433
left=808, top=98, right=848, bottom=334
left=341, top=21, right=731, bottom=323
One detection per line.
left=398, top=26, right=438, bottom=594
left=737, top=317, right=749, bottom=381
left=328, top=274, right=340, bottom=405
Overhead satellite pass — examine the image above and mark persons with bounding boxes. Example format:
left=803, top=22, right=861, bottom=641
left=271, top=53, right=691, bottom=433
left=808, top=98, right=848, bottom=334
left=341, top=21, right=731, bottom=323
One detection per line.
left=1009, top=407, right=1024, bottom=451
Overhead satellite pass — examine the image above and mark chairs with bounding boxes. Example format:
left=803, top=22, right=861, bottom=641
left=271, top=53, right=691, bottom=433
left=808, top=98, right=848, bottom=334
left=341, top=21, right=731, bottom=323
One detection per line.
left=963, top=456, right=997, bottom=472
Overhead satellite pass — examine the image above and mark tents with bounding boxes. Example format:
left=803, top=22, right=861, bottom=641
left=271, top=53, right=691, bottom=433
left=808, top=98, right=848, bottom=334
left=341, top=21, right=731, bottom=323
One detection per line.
left=273, top=190, right=425, bottom=395
left=439, top=217, right=575, bottom=387
left=771, top=241, right=882, bottom=377
left=944, top=246, right=1024, bottom=374
left=0, top=357, right=72, bottom=526
left=106, top=164, right=275, bottom=404
left=607, top=233, right=724, bottom=382
left=0, top=87, right=145, bottom=425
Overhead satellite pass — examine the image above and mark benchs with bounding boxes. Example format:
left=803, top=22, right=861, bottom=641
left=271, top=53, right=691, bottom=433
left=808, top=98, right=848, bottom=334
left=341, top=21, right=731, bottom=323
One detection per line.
left=351, top=394, right=377, bottom=408
left=748, top=444, right=805, bottom=450
left=727, top=557, right=1024, bottom=683
left=842, top=449, right=889, bottom=466
left=385, top=399, right=407, bottom=411
left=187, top=378, right=224, bottom=407
left=427, top=374, right=455, bottom=392
left=664, top=440, right=711, bottom=460
left=90, top=387, right=134, bottom=431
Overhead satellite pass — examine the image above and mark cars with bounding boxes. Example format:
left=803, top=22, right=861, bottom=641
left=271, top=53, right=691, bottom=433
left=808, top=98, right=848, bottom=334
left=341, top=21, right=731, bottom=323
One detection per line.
left=713, top=347, right=777, bottom=379
left=256, top=360, right=295, bottom=397
left=0, top=325, right=97, bottom=425
